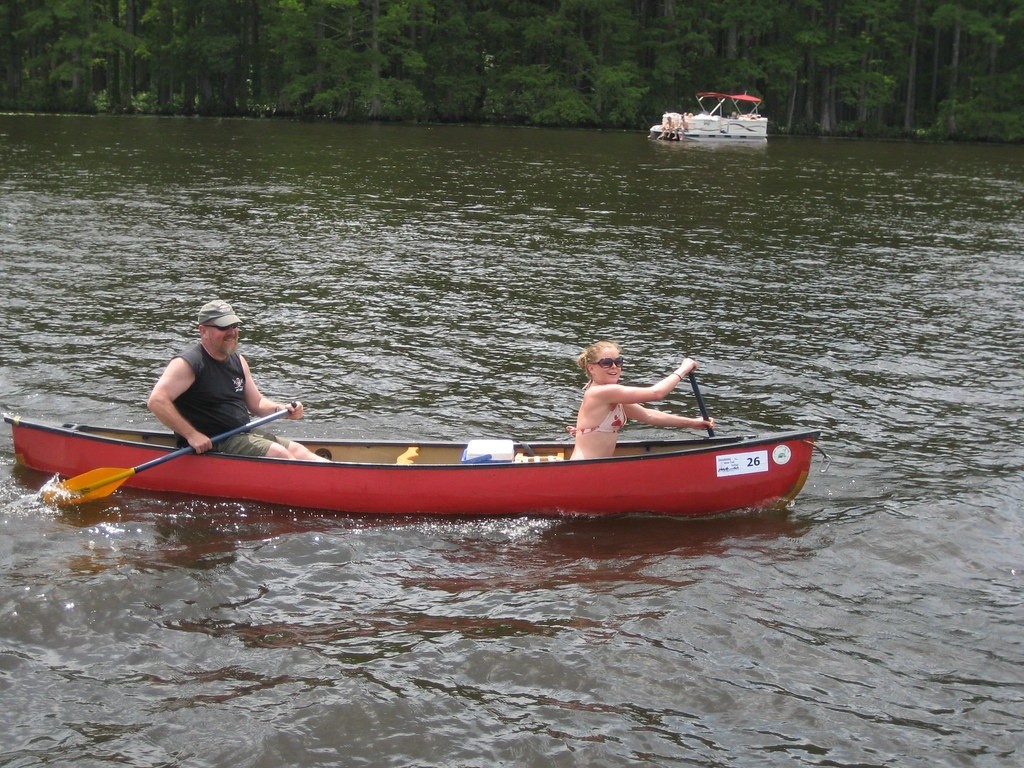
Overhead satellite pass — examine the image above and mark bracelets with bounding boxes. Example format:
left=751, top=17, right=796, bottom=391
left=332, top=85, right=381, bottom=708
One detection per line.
left=671, top=372, right=682, bottom=382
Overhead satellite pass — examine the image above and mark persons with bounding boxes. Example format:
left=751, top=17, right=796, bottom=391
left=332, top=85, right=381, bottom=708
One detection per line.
left=662, top=116, right=674, bottom=138
left=148, top=300, right=338, bottom=463
left=673, top=116, right=688, bottom=140
left=570, top=341, right=716, bottom=461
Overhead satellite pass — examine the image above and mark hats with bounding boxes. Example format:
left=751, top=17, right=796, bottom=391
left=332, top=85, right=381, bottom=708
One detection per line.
left=198, top=299, right=243, bottom=327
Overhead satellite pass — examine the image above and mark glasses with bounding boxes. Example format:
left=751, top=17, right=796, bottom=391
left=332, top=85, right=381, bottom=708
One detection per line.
left=588, top=356, right=624, bottom=368
left=203, top=322, right=238, bottom=331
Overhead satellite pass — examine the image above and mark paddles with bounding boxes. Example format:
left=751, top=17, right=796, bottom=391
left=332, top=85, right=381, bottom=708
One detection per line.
left=41, top=395, right=303, bottom=512
left=686, top=364, right=720, bottom=443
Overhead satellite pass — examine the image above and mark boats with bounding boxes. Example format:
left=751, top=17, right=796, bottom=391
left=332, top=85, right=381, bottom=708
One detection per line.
left=646, top=93, right=771, bottom=140
left=2, top=414, right=824, bottom=521
